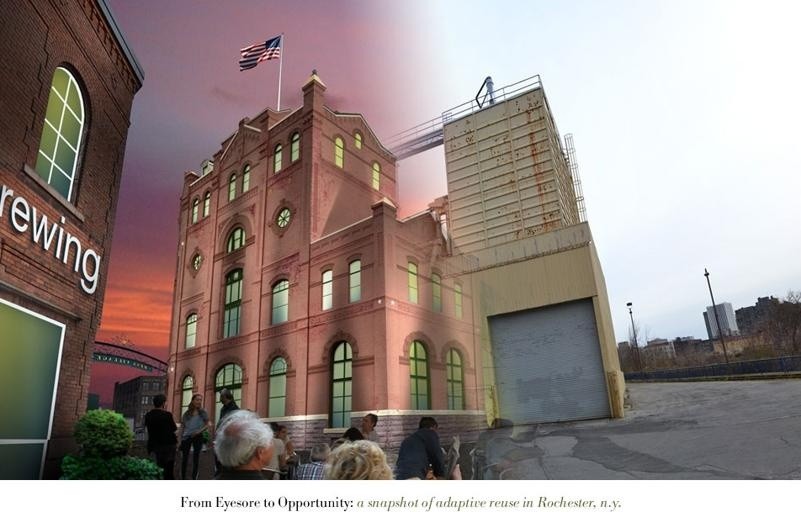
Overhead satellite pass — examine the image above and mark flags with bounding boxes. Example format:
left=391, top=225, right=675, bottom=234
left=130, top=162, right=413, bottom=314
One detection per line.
left=239, top=36, right=281, bottom=72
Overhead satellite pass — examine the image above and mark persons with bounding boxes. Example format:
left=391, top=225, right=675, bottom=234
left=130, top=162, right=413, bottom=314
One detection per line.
left=211, top=409, right=273, bottom=479
left=295, top=443, right=331, bottom=480
left=214, top=388, right=240, bottom=477
left=394, top=417, right=462, bottom=480
left=261, top=421, right=298, bottom=479
left=324, top=439, right=394, bottom=479
left=145, top=394, right=177, bottom=480
left=177, top=394, right=210, bottom=479
left=360, top=413, right=380, bottom=442
left=330, top=427, right=364, bottom=451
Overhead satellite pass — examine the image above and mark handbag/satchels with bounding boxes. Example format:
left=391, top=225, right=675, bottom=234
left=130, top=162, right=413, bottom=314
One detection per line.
left=203, top=427, right=209, bottom=444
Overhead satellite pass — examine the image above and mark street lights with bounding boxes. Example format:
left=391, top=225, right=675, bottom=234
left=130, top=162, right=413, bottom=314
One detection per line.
left=626, top=302, right=642, bottom=369
left=704, top=267, right=729, bottom=363
